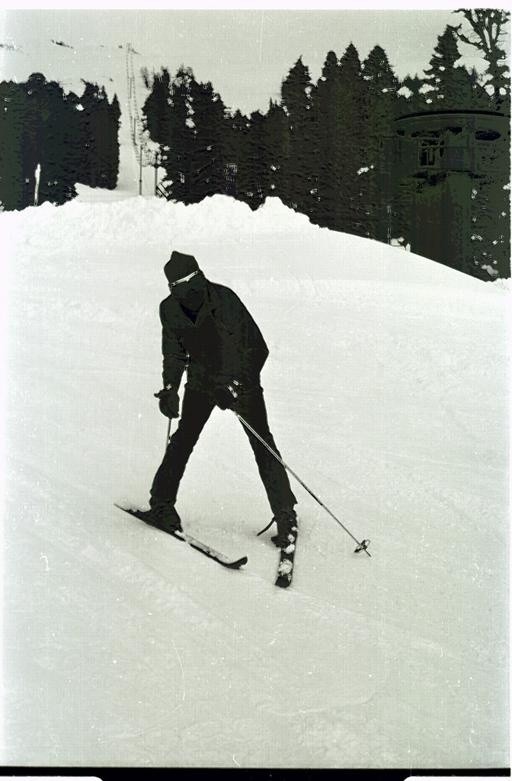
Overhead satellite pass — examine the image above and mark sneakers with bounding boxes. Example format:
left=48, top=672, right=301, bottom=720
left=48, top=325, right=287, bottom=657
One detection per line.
left=273, top=511, right=299, bottom=546
left=150, top=500, right=181, bottom=529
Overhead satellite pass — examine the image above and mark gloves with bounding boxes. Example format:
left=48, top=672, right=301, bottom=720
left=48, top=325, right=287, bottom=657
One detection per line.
left=207, top=377, right=244, bottom=410
left=154, top=386, right=181, bottom=420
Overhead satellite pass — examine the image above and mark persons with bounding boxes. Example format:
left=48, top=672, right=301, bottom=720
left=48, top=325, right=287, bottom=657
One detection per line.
left=150, top=251, right=298, bottom=547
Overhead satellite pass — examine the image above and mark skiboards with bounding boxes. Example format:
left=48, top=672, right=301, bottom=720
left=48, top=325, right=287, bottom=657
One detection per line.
left=114, top=498, right=296, bottom=590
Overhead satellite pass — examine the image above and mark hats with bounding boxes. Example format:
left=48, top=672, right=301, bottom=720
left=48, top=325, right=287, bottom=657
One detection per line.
left=163, top=252, right=200, bottom=285
